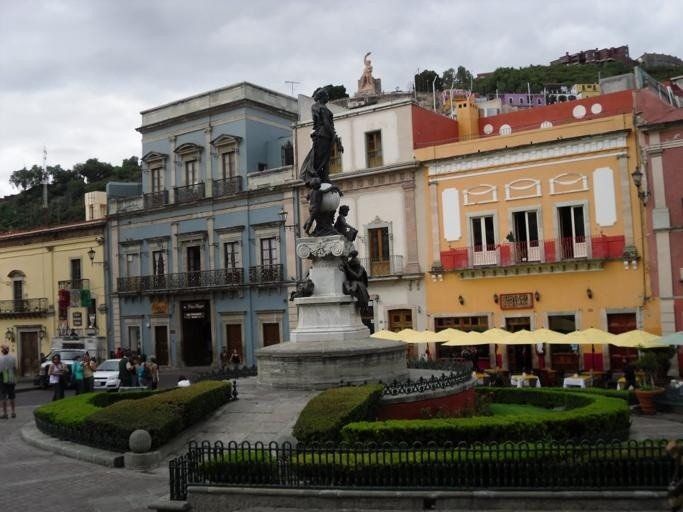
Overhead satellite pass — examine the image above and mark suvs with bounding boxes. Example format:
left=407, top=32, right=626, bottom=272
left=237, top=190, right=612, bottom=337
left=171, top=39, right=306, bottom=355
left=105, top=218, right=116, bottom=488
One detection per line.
left=39, top=349, right=90, bottom=386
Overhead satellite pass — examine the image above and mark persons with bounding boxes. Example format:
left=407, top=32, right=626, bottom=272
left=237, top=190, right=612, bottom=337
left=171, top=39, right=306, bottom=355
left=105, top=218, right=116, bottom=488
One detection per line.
left=220, top=346, right=230, bottom=374
left=343, top=256, right=368, bottom=297
left=0, top=345, right=17, bottom=418
left=334, top=205, right=358, bottom=242
left=289, top=279, right=314, bottom=302
left=41, top=348, right=160, bottom=401
left=310, top=87, right=343, bottom=184
left=303, top=178, right=322, bottom=236
left=359, top=52, right=375, bottom=92
left=229, top=348, right=241, bottom=369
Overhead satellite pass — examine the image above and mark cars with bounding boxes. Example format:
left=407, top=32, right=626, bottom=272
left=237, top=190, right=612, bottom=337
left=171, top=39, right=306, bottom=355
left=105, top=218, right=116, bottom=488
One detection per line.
left=93, top=359, right=121, bottom=390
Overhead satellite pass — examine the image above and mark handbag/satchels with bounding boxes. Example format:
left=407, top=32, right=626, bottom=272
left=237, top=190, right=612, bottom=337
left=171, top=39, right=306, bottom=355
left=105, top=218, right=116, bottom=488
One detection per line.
left=141, top=368, right=152, bottom=378
left=50, top=374, right=60, bottom=384
left=2, top=367, right=16, bottom=385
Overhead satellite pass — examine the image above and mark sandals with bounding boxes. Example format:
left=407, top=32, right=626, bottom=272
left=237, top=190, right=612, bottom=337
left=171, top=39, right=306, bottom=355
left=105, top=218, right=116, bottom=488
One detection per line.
left=0, top=413, right=16, bottom=419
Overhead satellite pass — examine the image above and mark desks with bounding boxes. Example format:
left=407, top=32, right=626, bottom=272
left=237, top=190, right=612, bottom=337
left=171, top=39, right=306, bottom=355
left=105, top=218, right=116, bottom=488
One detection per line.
left=475, top=368, right=626, bottom=391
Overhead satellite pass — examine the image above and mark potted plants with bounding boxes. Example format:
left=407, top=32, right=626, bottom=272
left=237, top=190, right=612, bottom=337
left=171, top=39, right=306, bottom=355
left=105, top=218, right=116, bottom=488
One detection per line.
left=630, top=344, right=665, bottom=416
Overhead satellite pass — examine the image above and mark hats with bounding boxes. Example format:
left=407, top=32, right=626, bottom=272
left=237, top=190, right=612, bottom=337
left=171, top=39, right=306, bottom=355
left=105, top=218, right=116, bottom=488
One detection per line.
left=1, top=346, right=10, bottom=353
